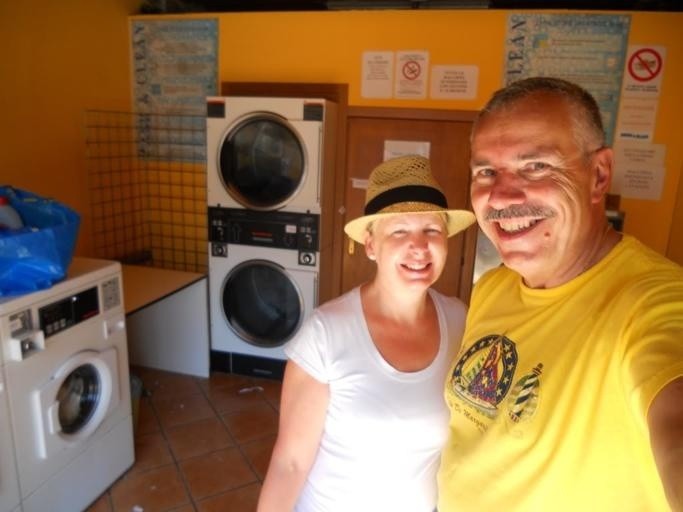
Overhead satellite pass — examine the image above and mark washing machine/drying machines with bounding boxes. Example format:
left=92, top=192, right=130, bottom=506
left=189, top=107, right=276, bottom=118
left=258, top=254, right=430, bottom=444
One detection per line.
left=0, top=256, right=136, bottom=512
left=205, top=95, right=337, bottom=384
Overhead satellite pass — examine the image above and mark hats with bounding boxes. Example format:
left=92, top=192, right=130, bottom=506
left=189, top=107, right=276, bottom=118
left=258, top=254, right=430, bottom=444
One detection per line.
left=343, top=154, right=477, bottom=246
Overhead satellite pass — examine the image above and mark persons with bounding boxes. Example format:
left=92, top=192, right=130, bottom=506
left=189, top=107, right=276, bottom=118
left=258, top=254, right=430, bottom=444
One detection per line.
left=441, top=76, right=682, bottom=512
left=252, top=153, right=480, bottom=512
